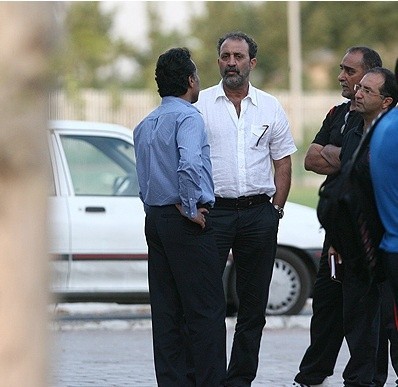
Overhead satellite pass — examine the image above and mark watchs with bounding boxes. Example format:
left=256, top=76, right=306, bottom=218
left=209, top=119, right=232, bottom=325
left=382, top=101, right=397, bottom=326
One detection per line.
left=273, top=203, right=284, bottom=218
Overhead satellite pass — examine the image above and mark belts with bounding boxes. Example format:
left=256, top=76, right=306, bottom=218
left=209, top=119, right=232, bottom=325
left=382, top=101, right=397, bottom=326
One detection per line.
left=209, top=193, right=270, bottom=209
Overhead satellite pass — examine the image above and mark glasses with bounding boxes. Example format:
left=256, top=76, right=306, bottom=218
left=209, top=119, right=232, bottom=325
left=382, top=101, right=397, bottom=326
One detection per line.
left=353, top=83, right=382, bottom=97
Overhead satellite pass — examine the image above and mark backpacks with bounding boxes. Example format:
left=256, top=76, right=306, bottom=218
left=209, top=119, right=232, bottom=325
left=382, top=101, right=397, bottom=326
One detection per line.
left=317, top=155, right=386, bottom=259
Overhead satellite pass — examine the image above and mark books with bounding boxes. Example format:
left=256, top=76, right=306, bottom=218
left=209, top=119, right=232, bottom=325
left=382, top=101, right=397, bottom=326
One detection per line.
left=330, top=254, right=343, bottom=283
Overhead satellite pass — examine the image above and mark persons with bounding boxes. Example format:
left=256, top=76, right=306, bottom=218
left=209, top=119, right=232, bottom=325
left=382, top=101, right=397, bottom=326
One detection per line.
left=369, top=98, right=398, bottom=386
left=134, top=47, right=227, bottom=387
left=294, top=47, right=381, bottom=387
left=338, top=67, right=397, bottom=386
left=189, top=31, right=297, bottom=387
